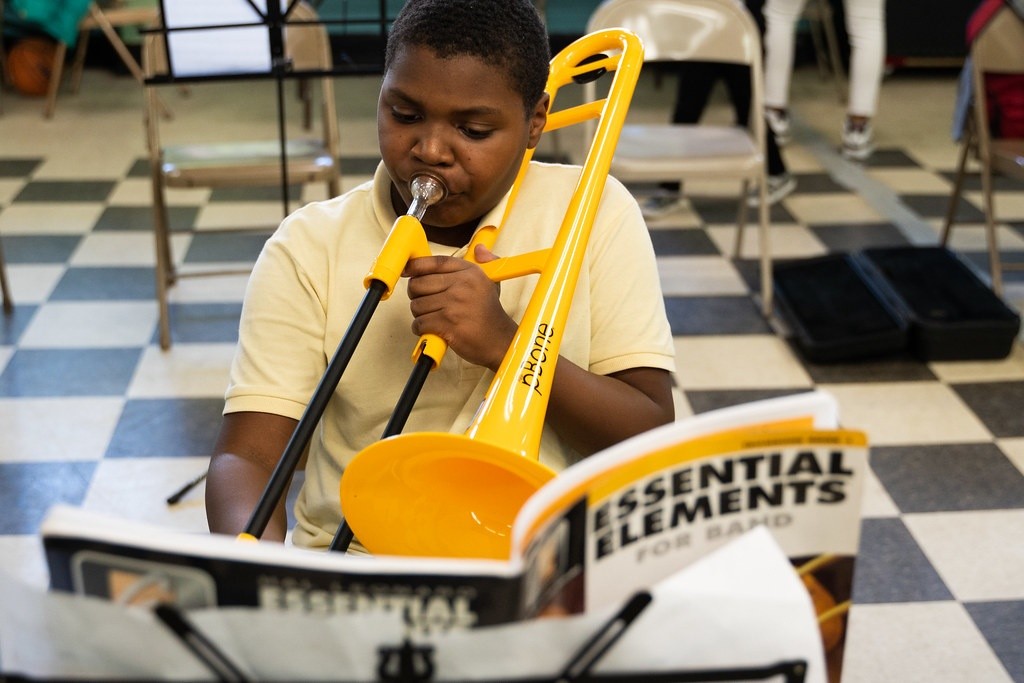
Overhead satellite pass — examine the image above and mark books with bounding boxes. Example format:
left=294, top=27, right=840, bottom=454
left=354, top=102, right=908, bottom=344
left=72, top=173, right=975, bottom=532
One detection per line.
left=36, top=390, right=865, bottom=683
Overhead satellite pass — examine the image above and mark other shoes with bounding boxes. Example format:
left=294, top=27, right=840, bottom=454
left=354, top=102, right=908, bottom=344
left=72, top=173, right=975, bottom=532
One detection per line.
left=640, top=196, right=682, bottom=216
left=765, top=113, right=791, bottom=145
left=838, top=125, right=878, bottom=159
left=746, top=173, right=795, bottom=207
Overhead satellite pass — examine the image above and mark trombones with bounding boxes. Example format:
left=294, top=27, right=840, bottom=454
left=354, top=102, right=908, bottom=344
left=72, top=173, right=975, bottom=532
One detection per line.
left=227, top=28, right=643, bottom=562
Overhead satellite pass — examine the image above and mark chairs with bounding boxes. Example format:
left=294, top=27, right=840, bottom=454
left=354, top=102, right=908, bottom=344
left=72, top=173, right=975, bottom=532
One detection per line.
left=144, top=0, right=342, bottom=352
left=44, top=0, right=191, bottom=122
left=938, top=0, right=1024, bottom=297
left=588, top=0, right=777, bottom=320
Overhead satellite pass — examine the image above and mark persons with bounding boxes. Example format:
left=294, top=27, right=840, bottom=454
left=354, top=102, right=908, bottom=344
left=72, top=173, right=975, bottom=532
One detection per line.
left=207, top=0, right=676, bottom=561
left=629, top=0, right=800, bottom=221
left=762, top=0, right=887, bottom=163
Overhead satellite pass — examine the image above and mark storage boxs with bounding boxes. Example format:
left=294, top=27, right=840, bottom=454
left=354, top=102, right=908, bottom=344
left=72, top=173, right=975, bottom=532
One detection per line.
left=775, top=245, right=1022, bottom=366
